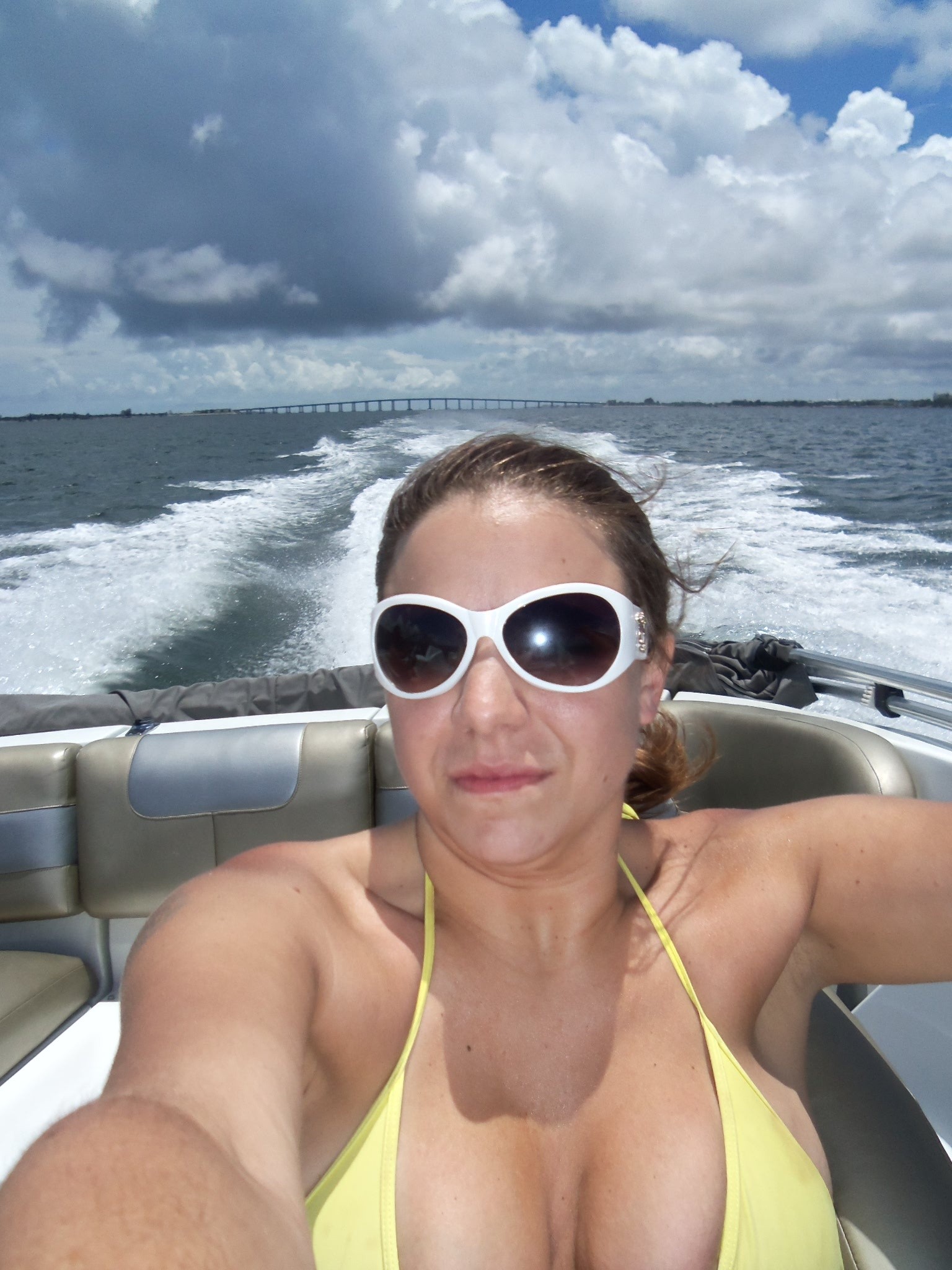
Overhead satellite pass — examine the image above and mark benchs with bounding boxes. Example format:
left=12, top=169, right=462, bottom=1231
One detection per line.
left=0, top=705, right=952, bottom=1270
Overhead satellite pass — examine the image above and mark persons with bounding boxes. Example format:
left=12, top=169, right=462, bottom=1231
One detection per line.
left=0, top=434, right=952, bottom=1270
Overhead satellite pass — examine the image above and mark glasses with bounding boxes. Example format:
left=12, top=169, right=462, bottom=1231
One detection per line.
left=366, top=582, right=650, bottom=697
